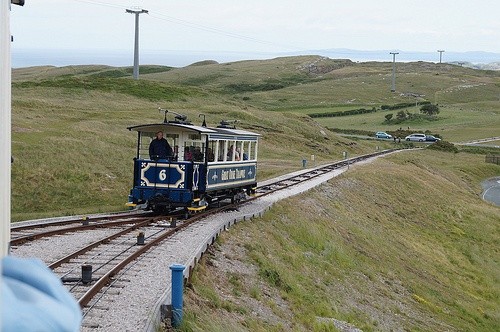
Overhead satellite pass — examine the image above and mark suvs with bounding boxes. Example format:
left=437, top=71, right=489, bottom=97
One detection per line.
left=376, top=132, right=393, bottom=139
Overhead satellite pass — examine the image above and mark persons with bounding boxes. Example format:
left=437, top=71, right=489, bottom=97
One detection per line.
left=175, top=145, right=214, bottom=161
left=394, top=134, right=397, bottom=142
left=0, top=256, right=82, bottom=332
left=222, top=145, right=248, bottom=161
left=148, top=131, right=172, bottom=163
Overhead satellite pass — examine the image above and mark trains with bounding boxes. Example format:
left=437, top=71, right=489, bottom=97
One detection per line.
left=124, top=106, right=262, bottom=220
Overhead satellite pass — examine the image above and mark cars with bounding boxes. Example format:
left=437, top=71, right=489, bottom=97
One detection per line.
left=425, top=135, right=442, bottom=142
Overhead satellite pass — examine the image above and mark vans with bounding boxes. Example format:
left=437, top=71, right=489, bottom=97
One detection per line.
left=405, top=133, right=426, bottom=142
